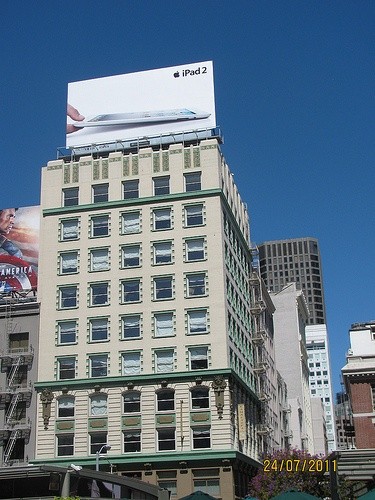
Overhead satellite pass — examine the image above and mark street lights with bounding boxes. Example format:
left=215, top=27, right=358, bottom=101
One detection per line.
left=97, top=454, right=116, bottom=499
left=96, top=444, right=111, bottom=474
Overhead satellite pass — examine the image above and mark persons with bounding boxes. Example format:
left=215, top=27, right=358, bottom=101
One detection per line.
left=0, top=208, right=23, bottom=259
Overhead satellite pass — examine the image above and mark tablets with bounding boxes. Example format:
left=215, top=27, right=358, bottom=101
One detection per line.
left=74, top=106, right=212, bottom=127
